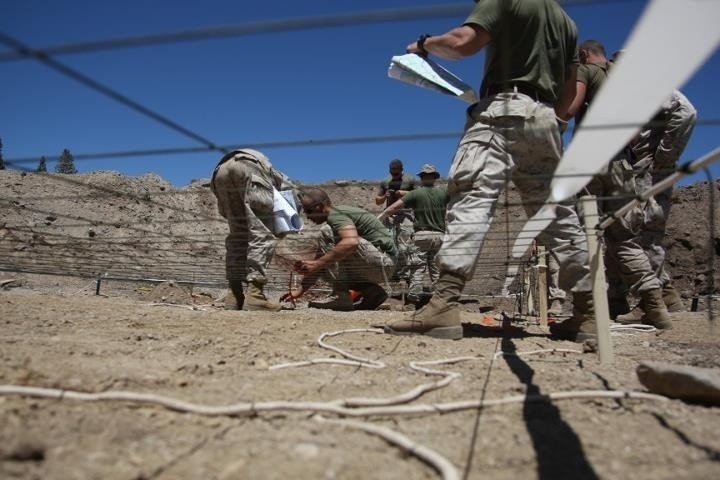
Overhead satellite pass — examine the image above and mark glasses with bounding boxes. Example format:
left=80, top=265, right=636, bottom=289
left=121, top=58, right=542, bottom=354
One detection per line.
left=303, top=203, right=320, bottom=215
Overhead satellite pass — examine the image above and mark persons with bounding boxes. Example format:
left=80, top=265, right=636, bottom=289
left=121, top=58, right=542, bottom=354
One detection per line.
left=383, top=0, right=600, bottom=346
left=544, top=252, right=567, bottom=318
left=605, top=50, right=699, bottom=325
left=564, top=39, right=677, bottom=331
left=374, top=163, right=452, bottom=311
left=374, top=159, right=419, bottom=288
left=278, top=188, right=400, bottom=311
left=210, top=147, right=305, bottom=313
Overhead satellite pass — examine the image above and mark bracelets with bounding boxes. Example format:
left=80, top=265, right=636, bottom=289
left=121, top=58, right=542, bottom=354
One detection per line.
left=416, top=33, right=432, bottom=54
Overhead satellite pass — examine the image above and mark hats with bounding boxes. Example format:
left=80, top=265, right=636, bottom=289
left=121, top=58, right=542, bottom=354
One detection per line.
left=416, top=164, right=440, bottom=179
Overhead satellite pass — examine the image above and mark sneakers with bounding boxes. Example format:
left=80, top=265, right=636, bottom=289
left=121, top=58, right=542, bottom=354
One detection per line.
left=354, top=285, right=389, bottom=310
left=396, top=301, right=416, bottom=310
left=309, top=288, right=353, bottom=310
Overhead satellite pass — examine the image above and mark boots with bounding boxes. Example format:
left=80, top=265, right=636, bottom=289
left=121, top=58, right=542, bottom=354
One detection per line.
left=616, top=285, right=684, bottom=331
left=384, top=273, right=466, bottom=340
left=550, top=292, right=597, bottom=342
left=225, top=281, right=283, bottom=310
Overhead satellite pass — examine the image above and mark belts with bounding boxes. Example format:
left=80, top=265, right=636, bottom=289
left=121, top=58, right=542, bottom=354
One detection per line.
left=481, top=83, right=549, bottom=103
